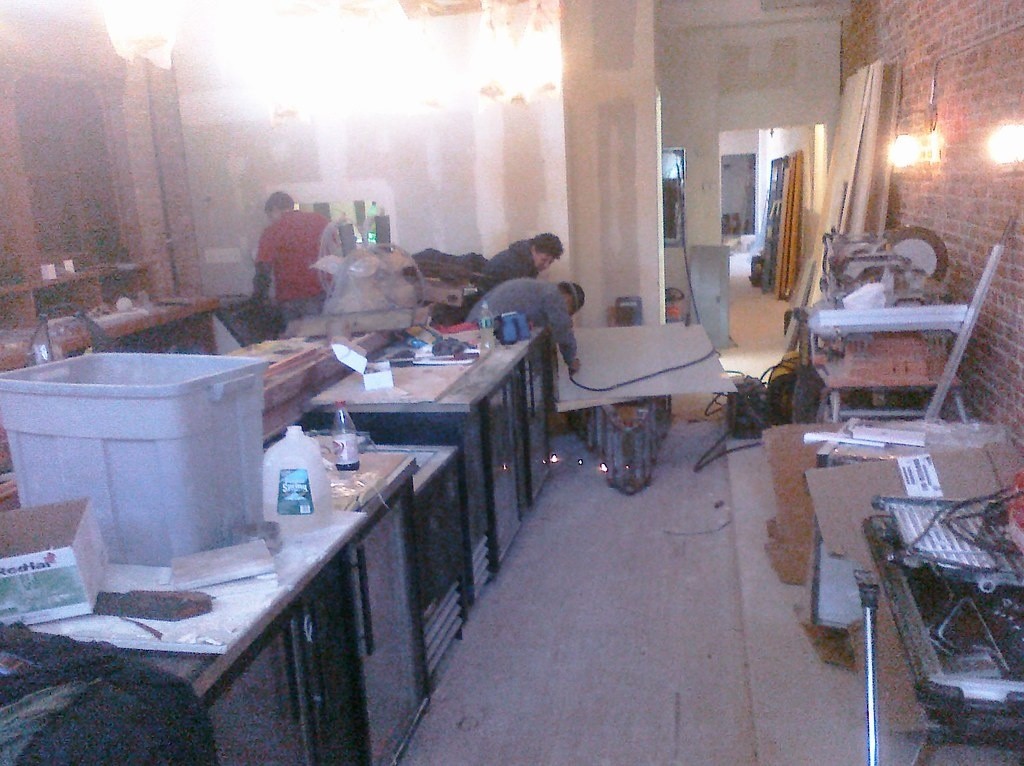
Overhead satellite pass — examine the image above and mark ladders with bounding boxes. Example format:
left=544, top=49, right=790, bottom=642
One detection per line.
left=568, top=393, right=672, bottom=496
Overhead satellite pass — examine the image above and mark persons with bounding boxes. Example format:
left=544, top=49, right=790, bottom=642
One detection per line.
left=477, top=233, right=564, bottom=296
left=464, top=276, right=586, bottom=374
left=251, top=191, right=337, bottom=328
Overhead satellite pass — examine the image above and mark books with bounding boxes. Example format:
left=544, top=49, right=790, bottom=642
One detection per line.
left=171, top=538, right=276, bottom=588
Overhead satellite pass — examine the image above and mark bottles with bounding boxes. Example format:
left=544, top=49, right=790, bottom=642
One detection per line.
left=478, top=305, right=494, bottom=348
left=367, top=201, right=378, bottom=242
left=331, top=401, right=359, bottom=471
left=265, top=426, right=332, bottom=534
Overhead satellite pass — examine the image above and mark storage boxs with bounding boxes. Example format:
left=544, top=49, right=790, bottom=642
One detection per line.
left=0, top=353, right=268, bottom=565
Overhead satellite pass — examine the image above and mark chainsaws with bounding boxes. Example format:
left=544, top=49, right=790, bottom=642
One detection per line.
left=818, top=223, right=950, bottom=309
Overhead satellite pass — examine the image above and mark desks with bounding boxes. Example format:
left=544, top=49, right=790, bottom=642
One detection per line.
left=812, top=336, right=971, bottom=424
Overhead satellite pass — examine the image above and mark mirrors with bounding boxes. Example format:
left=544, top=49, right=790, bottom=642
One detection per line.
left=661, top=146, right=687, bottom=247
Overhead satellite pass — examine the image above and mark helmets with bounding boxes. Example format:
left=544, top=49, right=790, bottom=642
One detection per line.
left=559, top=282, right=585, bottom=315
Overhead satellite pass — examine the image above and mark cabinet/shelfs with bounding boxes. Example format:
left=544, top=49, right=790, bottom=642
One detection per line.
left=307, top=325, right=555, bottom=576
left=21, top=449, right=427, bottom=766
left=0, top=0, right=221, bottom=472
left=364, top=442, right=470, bottom=699
